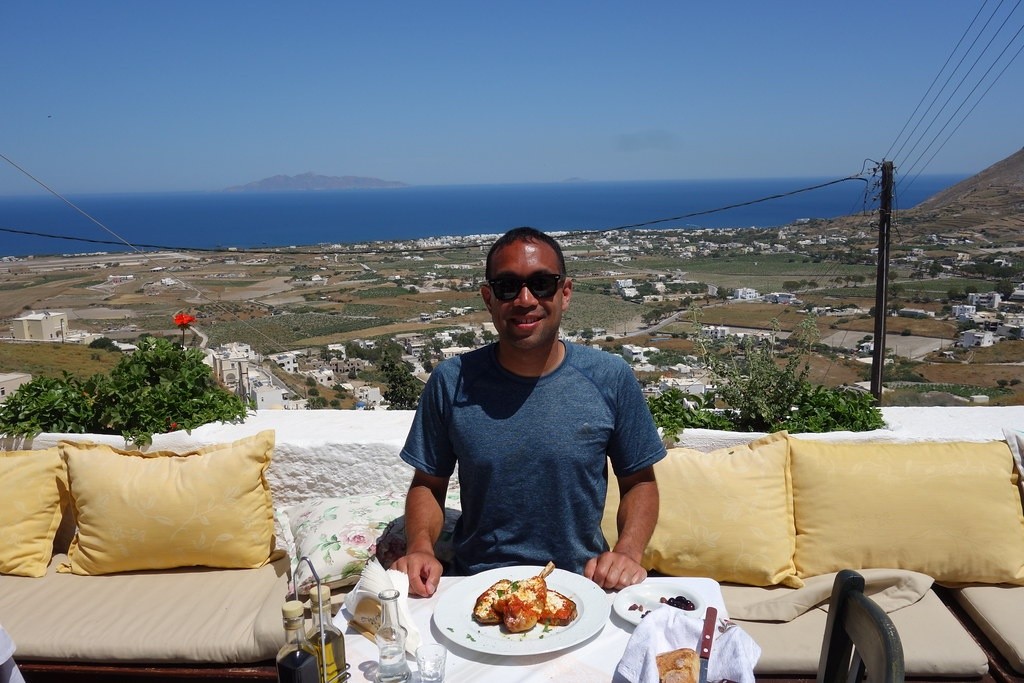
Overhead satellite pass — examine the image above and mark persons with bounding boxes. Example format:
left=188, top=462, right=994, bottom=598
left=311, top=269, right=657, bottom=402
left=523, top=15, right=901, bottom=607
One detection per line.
left=389, top=227, right=668, bottom=597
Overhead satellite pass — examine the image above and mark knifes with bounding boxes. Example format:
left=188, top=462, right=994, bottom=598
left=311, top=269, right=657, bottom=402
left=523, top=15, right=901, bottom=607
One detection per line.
left=699, top=606, right=717, bottom=683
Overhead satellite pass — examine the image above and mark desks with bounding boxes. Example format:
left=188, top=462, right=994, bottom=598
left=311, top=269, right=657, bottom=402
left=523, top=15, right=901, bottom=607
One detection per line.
left=314, top=575, right=747, bottom=683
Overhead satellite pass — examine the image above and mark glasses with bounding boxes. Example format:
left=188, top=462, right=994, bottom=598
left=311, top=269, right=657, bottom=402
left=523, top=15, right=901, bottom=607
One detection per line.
left=486, top=273, right=565, bottom=301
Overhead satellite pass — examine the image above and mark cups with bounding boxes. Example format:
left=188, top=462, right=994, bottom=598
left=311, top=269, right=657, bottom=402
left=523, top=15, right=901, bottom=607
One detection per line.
left=415, top=643, right=447, bottom=683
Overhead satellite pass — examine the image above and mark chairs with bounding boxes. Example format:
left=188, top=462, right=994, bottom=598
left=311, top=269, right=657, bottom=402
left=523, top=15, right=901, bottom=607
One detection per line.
left=812, top=569, right=902, bottom=683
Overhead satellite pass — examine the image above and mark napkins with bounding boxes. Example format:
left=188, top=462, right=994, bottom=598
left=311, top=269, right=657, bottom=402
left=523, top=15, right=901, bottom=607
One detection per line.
left=342, top=557, right=424, bottom=658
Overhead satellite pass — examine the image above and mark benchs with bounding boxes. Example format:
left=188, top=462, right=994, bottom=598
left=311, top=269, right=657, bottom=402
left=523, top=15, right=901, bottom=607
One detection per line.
left=0, top=556, right=1024, bottom=683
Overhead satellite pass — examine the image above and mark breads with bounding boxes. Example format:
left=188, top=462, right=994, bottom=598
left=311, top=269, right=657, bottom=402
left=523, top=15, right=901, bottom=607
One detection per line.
left=655, top=648, right=701, bottom=683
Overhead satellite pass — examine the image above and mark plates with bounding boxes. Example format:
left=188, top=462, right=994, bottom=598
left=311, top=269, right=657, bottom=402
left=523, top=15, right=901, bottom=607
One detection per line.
left=613, top=582, right=707, bottom=627
left=433, top=565, right=612, bottom=657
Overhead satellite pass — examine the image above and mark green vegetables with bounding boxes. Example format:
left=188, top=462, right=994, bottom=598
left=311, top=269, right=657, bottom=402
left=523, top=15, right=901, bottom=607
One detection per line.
left=446, top=580, right=552, bottom=641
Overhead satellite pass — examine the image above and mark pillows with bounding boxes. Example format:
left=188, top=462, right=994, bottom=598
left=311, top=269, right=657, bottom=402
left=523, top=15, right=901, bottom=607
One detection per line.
left=57, top=431, right=287, bottom=577
left=599, top=430, right=806, bottom=588
left=275, top=484, right=466, bottom=600
left=0, top=442, right=75, bottom=579
left=782, top=438, right=1024, bottom=584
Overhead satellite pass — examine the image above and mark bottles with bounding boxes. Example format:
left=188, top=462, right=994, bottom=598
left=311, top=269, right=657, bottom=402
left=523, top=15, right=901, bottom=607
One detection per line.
left=305, top=585, right=348, bottom=683
left=374, top=589, right=413, bottom=683
left=275, top=599, right=323, bottom=683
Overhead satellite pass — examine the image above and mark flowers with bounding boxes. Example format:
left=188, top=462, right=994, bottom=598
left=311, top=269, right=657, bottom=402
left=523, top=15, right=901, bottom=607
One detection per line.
left=91, top=309, right=240, bottom=435
